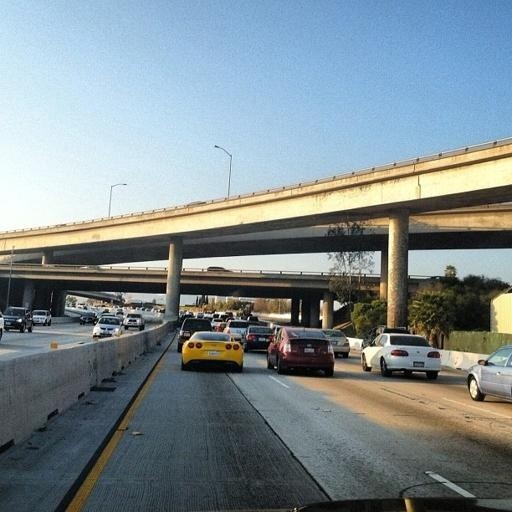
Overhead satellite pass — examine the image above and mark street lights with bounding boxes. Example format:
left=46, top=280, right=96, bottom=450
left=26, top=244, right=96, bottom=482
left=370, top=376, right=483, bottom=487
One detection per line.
left=214, top=145, right=233, bottom=199
left=108, top=183, right=127, bottom=219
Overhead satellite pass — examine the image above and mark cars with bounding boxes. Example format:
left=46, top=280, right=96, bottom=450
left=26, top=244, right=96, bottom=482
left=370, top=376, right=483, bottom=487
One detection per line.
left=287, top=493, right=512, bottom=512
left=66, top=302, right=165, bottom=344
left=465, top=344, right=512, bottom=405
left=176, top=306, right=441, bottom=381
left=31, top=309, right=52, bottom=328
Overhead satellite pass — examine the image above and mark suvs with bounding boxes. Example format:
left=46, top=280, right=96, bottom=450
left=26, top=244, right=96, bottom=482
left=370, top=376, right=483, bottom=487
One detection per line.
left=3, top=305, right=35, bottom=333
left=1, top=312, right=6, bottom=342
left=207, top=266, right=232, bottom=272
